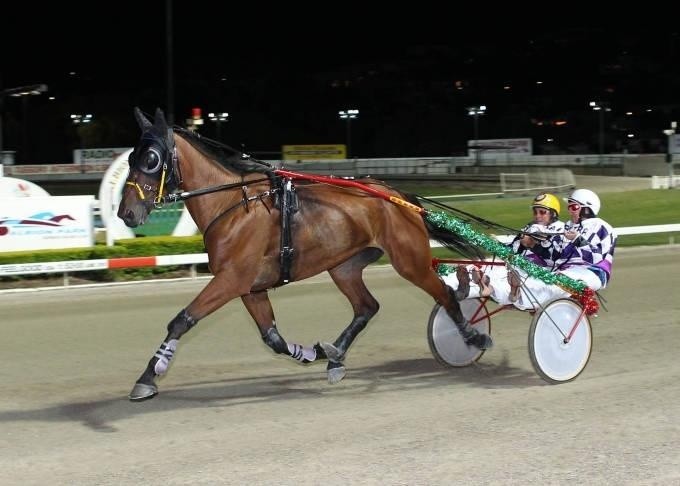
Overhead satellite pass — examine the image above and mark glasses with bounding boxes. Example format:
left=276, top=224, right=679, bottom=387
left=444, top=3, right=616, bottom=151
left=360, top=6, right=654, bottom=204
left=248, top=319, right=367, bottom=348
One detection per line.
left=567, top=202, right=581, bottom=212
left=533, top=209, right=546, bottom=215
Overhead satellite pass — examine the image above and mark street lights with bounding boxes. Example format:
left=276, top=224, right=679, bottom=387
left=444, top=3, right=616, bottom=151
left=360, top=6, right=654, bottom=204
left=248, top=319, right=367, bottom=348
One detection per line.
left=465, top=104, right=489, bottom=141
left=339, top=107, right=360, bottom=155
left=70, top=111, right=95, bottom=147
left=588, top=100, right=610, bottom=153
left=207, top=107, right=233, bottom=142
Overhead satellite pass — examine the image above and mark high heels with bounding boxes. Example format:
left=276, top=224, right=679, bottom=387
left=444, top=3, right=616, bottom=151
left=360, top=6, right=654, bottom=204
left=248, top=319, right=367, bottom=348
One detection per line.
left=472, top=266, right=490, bottom=298
left=507, top=270, right=521, bottom=302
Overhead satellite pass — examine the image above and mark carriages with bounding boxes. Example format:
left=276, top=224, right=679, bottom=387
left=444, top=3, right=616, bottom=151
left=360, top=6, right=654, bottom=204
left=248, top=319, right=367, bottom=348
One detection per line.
left=115, top=106, right=594, bottom=402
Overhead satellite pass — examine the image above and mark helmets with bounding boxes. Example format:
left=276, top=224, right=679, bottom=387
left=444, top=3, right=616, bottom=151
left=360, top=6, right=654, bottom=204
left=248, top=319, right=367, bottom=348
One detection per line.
left=530, top=192, right=561, bottom=217
left=567, top=189, right=601, bottom=217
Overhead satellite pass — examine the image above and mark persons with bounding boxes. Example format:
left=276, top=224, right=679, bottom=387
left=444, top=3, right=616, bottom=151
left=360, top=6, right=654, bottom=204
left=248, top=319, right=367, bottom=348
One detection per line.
left=470, top=189, right=620, bottom=303
left=452, top=192, right=566, bottom=298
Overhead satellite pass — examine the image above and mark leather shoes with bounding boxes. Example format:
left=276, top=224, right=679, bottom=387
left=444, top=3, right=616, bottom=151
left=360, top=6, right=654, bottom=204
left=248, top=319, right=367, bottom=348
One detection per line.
left=453, top=267, right=470, bottom=301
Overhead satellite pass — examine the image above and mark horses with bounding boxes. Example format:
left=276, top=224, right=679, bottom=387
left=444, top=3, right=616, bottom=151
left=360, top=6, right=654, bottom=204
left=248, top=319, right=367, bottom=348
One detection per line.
left=117, top=106, right=491, bottom=402
